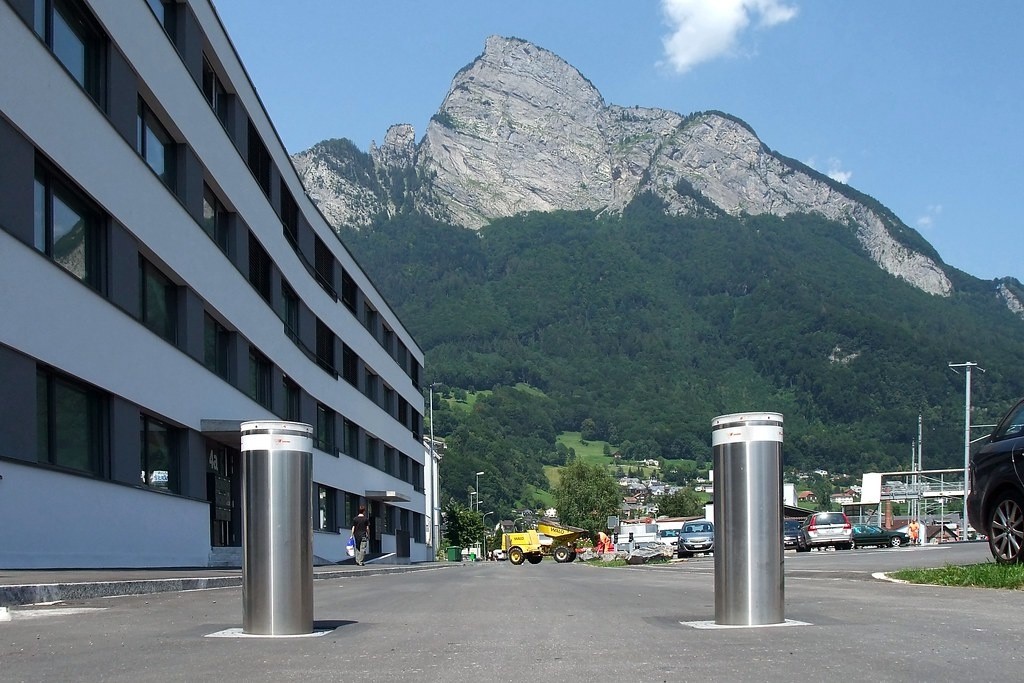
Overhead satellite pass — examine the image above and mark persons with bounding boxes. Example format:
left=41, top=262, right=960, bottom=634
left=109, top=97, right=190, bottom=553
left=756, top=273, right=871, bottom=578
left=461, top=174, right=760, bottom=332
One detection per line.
left=594, top=530, right=610, bottom=560
left=908, top=519, right=920, bottom=547
left=350, top=506, right=370, bottom=566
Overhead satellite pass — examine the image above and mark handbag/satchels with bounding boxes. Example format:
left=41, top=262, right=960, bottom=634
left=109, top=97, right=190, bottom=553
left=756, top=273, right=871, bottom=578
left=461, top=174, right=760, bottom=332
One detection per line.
left=346, top=538, right=355, bottom=557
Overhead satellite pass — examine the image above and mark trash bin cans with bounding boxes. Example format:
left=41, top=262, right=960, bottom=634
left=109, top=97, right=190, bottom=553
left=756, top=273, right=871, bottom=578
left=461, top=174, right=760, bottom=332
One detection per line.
left=470, top=552, right=476, bottom=561
left=445, top=546, right=462, bottom=562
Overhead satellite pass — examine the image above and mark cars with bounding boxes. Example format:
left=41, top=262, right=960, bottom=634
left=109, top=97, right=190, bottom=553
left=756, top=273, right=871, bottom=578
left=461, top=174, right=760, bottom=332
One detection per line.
left=852, top=524, right=910, bottom=548
left=677, top=520, right=716, bottom=557
left=656, top=526, right=681, bottom=555
left=782, top=518, right=802, bottom=550
left=965, top=395, right=1024, bottom=567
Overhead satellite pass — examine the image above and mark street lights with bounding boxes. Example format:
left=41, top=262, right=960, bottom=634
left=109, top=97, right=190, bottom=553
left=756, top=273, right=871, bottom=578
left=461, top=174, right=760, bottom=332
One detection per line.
left=470, top=500, right=484, bottom=512
left=470, top=491, right=477, bottom=511
left=476, top=471, right=485, bottom=513
left=429, top=382, right=442, bottom=558
left=483, top=511, right=494, bottom=559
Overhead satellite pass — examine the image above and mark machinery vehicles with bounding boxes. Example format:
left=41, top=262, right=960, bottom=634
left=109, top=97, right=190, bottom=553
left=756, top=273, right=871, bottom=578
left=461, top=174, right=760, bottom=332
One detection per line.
left=501, top=520, right=589, bottom=565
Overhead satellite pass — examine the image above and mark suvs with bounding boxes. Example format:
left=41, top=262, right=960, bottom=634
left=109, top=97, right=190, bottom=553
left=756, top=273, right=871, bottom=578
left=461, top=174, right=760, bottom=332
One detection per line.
left=799, top=510, right=852, bottom=552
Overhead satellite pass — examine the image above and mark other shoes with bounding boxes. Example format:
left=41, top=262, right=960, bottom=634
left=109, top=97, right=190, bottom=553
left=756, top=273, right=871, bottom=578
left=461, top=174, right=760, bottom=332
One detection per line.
left=357, top=563, right=365, bottom=566
